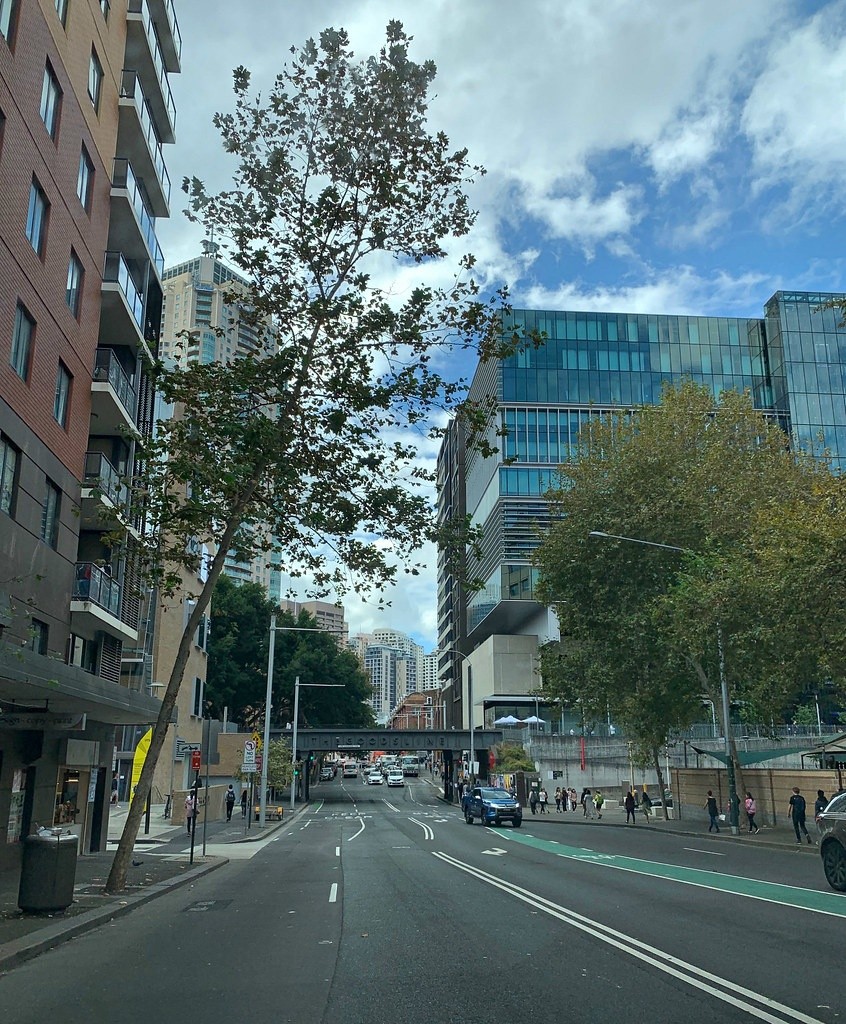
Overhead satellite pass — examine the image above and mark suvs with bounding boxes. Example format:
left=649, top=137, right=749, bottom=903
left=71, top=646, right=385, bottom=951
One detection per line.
left=386, top=769, right=405, bottom=787
left=814, top=790, right=846, bottom=892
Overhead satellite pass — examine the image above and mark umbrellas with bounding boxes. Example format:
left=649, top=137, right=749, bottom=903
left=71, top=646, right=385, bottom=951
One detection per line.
left=494, top=715, right=546, bottom=729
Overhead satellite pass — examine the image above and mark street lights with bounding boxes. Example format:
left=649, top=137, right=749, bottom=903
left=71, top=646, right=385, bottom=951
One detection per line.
left=436, top=649, right=474, bottom=785
left=588, top=530, right=741, bottom=836
left=258, top=614, right=349, bottom=828
left=289, top=676, right=346, bottom=809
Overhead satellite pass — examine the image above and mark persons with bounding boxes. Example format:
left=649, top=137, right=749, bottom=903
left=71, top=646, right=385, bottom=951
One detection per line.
left=610, top=724, right=616, bottom=737
left=569, top=728, right=575, bottom=736
left=640, top=792, right=652, bottom=823
left=528, top=787, right=550, bottom=815
left=476, top=781, right=481, bottom=787
left=744, top=793, right=760, bottom=834
left=703, top=791, right=720, bottom=832
left=787, top=787, right=812, bottom=845
left=580, top=788, right=603, bottom=820
left=727, top=794, right=740, bottom=824
left=110, top=773, right=121, bottom=808
left=814, top=790, right=828, bottom=820
left=624, top=792, right=636, bottom=824
left=459, top=776, right=468, bottom=797
left=224, top=784, right=235, bottom=822
left=554, top=786, right=577, bottom=813
left=184, top=790, right=200, bottom=836
left=435, top=765, right=438, bottom=775
left=239, top=790, right=250, bottom=818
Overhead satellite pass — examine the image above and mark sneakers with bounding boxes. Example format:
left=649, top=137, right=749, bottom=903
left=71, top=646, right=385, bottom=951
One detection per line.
left=747, top=831, right=753, bottom=833
left=754, top=828, right=760, bottom=835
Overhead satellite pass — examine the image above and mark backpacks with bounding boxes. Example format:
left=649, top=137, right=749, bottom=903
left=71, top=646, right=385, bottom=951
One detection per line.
left=227, top=790, right=235, bottom=802
left=794, top=796, right=805, bottom=815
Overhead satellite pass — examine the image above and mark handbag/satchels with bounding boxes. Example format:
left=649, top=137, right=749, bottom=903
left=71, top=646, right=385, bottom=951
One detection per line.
left=192, top=804, right=201, bottom=814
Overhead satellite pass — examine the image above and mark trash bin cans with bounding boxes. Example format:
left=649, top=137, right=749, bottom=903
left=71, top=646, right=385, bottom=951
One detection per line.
left=18, top=833, right=78, bottom=916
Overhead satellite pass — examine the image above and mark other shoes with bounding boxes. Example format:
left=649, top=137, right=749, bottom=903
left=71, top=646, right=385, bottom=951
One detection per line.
left=540, top=810, right=577, bottom=814
left=716, top=829, right=721, bottom=833
left=187, top=832, right=191, bottom=837
left=584, top=814, right=603, bottom=820
left=796, top=839, right=802, bottom=845
left=806, top=835, right=812, bottom=844
left=229, top=819, right=232, bottom=823
left=708, top=828, right=712, bottom=833
left=226, top=817, right=229, bottom=823
left=625, top=819, right=629, bottom=824
left=242, top=816, right=245, bottom=820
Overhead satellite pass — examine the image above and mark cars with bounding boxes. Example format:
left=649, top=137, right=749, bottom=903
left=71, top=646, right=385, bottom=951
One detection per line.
left=319, top=755, right=406, bottom=781
left=460, top=787, right=523, bottom=828
left=367, top=772, right=384, bottom=784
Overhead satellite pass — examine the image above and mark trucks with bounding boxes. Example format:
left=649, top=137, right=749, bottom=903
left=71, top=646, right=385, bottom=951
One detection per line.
left=400, top=755, right=420, bottom=777
